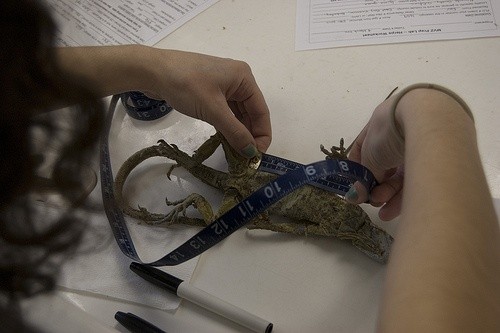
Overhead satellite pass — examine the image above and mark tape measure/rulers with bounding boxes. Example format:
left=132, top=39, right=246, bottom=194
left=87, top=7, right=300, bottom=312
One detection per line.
left=99, top=89, right=382, bottom=268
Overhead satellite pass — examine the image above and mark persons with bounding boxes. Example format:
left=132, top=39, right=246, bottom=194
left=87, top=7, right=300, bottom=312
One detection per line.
left=1, top=0, right=500, bottom=333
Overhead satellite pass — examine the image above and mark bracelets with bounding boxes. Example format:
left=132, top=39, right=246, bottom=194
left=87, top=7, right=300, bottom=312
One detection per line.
left=390, top=82, right=474, bottom=143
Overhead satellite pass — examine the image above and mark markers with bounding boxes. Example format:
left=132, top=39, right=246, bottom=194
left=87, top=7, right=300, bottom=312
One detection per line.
left=128, top=261, right=274, bottom=333
left=115, top=311, right=169, bottom=333
